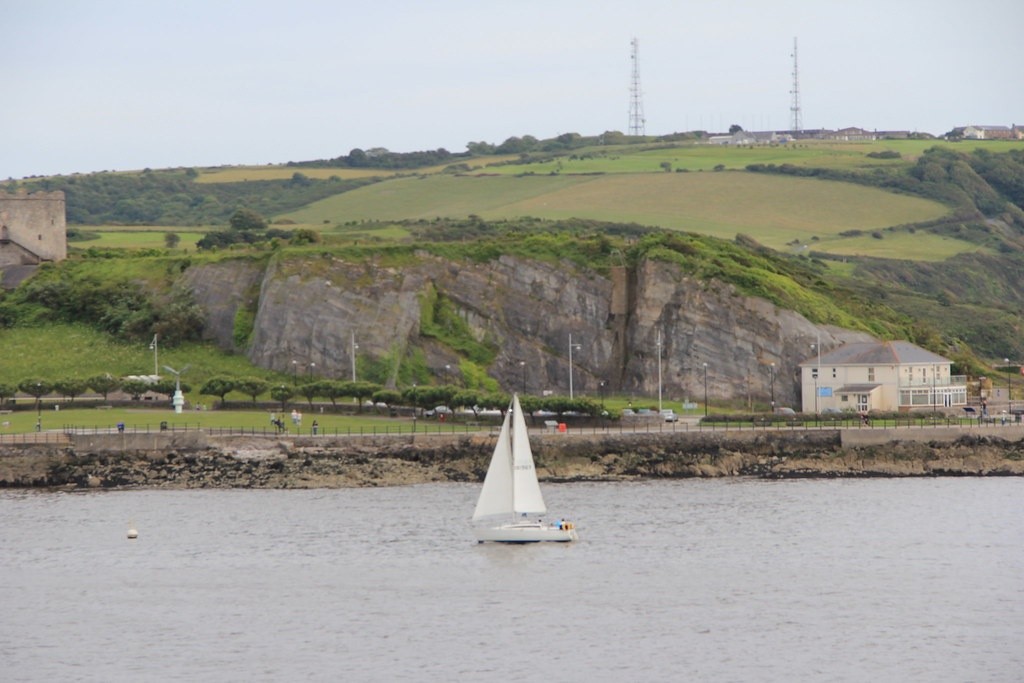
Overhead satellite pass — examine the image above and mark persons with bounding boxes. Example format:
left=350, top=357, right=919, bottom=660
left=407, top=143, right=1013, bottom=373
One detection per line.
left=558, top=518, right=567, bottom=530
left=858, top=411, right=873, bottom=427
left=537, top=519, right=542, bottom=528
left=311, top=417, right=320, bottom=437
left=194, top=400, right=207, bottom=412
left=291, top=409, right=304, bottom=425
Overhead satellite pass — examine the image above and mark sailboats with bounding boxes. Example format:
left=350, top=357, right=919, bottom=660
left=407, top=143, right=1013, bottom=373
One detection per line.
left=471, top=392, right=578, bottom=541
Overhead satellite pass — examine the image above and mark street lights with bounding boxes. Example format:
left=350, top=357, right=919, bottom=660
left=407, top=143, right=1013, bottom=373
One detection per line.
left=520, top=361, right=526, bottom=395
left=351, top=333, right=359, bottom=403
left=770, top=362, right=775, bottom=411
left=150, top=334, right=157, bottom=377
left=292, top=360, right=297, bottom=387
left=444, top=365, right=450, bottom=408
left=811, top=372, right=818, bottom=426
left=810, top=336, right=821, bottom=415
left=568, top=333, right=582, bottom=399
left=309, top=362, right=315, bottom=383
left=1004, top=358, right=1011, bottom=414
left=703, top=362, right=707, bottom=417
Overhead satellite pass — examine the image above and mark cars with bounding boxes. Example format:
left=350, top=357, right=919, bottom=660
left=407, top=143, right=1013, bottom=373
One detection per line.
left=621, top=407, right=679, bottom=423
left=425, top=405, right=453, bottom=417
left=772, top=406, right=881, bottom=415
